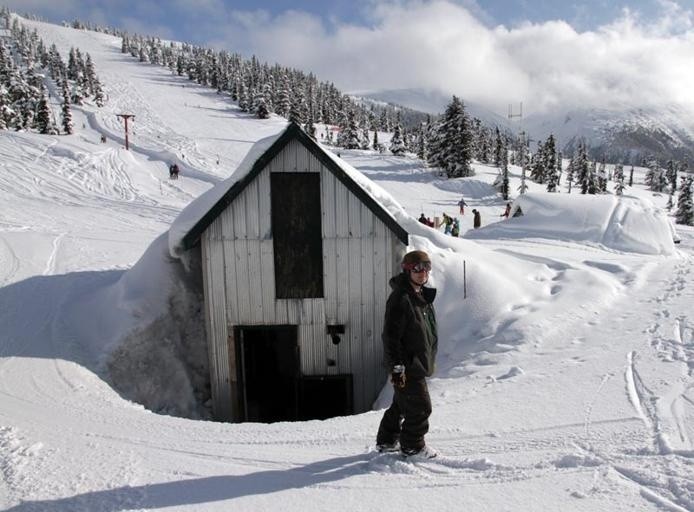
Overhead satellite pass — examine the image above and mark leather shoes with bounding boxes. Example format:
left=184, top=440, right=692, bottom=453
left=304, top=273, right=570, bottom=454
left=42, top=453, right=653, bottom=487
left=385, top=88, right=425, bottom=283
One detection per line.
left=390, top=364, right=406, bottom=389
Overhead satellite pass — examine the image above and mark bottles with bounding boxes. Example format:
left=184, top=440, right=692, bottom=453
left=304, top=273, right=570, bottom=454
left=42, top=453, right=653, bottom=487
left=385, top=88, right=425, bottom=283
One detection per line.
left=404, top=250, right=432, bottom=270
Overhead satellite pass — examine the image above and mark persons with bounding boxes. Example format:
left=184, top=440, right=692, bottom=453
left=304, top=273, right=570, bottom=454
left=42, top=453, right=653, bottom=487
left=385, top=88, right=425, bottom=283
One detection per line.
left=169, top=164, right=178, bottom=179
left=501, top=203, right=511, bottom=219
left=458, top=199, right=468, bottom=214
left=417, top=212, right=461, bottom=237
left=472, top=209, right=481, bottom=228
left=375, top=250, right=441, bottom=459
left=101, top=135, right=103, bottom=143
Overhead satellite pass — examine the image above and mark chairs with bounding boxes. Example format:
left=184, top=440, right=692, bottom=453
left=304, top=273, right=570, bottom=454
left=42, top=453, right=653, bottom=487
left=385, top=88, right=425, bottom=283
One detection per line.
left=401, top=444, right=438, bottom=460
left=376, top=440, right=402, bottom=453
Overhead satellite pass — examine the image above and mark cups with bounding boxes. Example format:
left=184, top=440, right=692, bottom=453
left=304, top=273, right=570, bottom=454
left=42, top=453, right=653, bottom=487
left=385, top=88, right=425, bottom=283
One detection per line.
left=410, top=260, right=432, bottom=273
